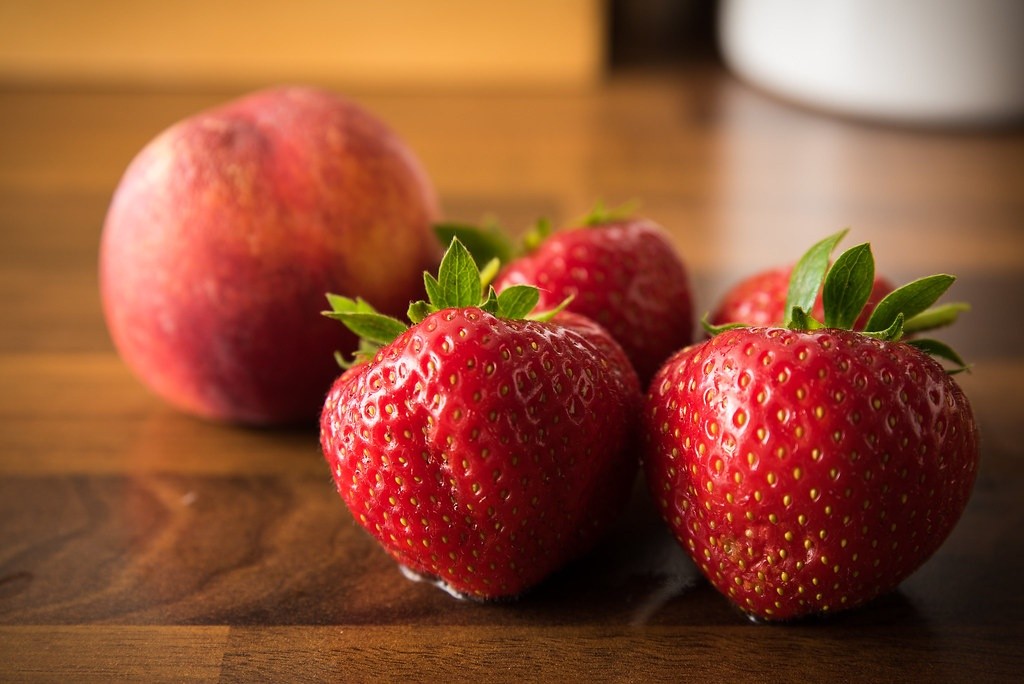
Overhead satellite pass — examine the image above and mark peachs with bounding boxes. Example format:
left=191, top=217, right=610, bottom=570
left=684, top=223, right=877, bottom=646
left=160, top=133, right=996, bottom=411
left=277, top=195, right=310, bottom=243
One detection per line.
left=100, top=85, right=445, bottom=424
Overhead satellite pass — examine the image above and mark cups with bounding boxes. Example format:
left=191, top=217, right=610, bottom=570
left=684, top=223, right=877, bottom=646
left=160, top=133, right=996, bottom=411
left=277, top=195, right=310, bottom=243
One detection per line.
left=720, top=0, right=1024, bottom=132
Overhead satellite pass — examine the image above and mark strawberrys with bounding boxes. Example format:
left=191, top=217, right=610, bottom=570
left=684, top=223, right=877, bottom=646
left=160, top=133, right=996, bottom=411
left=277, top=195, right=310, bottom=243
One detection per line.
left=316, top=198, right=980, bottom=625
